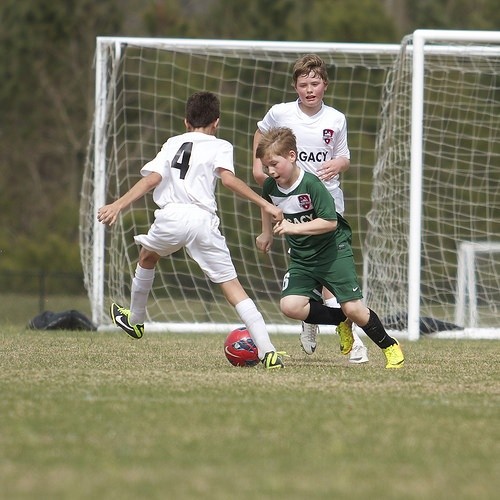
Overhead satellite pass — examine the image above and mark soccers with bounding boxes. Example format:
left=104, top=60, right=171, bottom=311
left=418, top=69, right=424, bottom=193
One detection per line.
left=224, top=327, right=260, bottom=367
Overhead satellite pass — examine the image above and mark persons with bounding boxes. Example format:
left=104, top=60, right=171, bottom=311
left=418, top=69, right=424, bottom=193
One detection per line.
left=253, top=126, right=405, bottom=367
left=99, top=90, right=285, bottom=367
left=249, top=52, right=369, bottom=364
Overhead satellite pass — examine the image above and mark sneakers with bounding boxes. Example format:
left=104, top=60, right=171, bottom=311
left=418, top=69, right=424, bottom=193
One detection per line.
left=259, top=350, right=290, bottom=370
left=109, top=302, right=144, bottom=340
left=381, top=336, right=405, bottom=369
left=300, top=319, right=320, bottom=356
left=336, top=318, right=354, bottom=354
left=350, top=343, right=369, bottom=364
